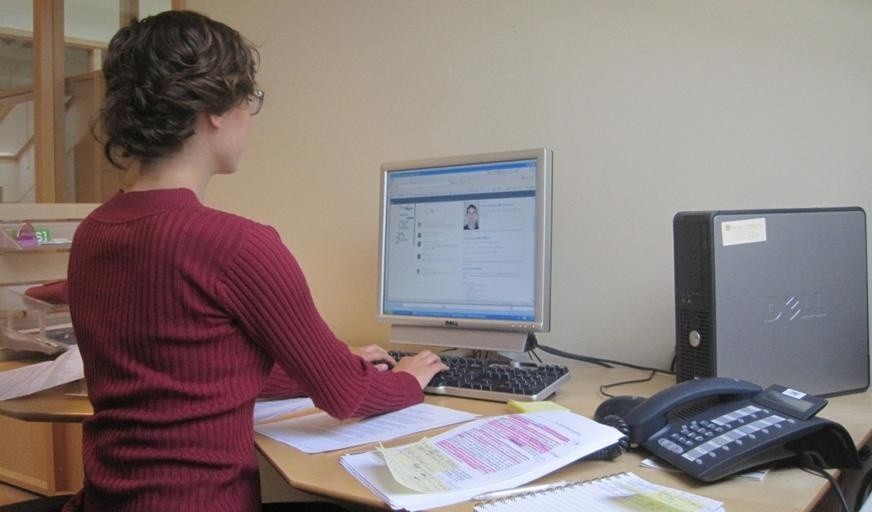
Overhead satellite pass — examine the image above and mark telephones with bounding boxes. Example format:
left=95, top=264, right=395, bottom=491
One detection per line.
left=624, top=378, right=863, bottom=482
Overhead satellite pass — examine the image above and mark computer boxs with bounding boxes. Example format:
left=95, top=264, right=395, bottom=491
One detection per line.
left=673, top=208, right=870, bottom=396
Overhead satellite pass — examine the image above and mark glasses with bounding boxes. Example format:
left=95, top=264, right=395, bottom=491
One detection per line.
left=245, top=88, right=264, bottom=116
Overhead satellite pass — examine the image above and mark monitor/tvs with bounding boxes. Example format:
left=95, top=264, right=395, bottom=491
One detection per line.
left=377, top=146, right=551, bottom=366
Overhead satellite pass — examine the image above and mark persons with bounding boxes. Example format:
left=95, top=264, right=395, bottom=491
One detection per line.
left=464, top=204, right=479, bottom=230
left=66, top=8, right=449, bottom=512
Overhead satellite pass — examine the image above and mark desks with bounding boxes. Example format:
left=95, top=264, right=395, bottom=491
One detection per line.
left=0, top=338, right=871, bottom=512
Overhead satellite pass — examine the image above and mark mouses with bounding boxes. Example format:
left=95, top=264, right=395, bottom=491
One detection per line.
left=593, top=395, right=647, bottom=424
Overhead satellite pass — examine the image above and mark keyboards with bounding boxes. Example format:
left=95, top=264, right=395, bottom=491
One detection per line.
left=367, top=350, right=570, bottom=402
